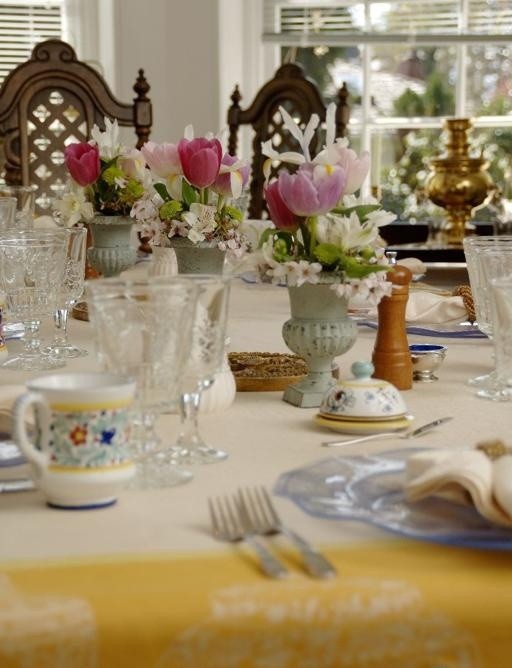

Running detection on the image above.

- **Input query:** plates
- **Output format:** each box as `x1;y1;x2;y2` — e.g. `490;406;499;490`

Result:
350;308;487;338
238;271;287;287
271;446;511;550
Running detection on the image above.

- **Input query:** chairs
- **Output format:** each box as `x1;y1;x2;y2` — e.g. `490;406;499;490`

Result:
1;39;156;218
220;62;348;222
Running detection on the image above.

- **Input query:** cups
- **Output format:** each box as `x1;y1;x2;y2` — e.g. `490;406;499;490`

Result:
10;372;137;512
11;227;88;361
474;246;512;401
82;276;194;490
463;235;512;388
0;238;67;372
145;273;233;466
1;187;39;230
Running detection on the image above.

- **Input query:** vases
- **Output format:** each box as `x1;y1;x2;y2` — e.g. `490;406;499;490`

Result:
281;285;354;408
166;238;225;275
425;116;490;246
83;213;136;276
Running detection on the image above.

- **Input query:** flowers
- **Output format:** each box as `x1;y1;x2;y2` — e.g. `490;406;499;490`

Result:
264;140;391;318
144;127;252;255
57;118;147;234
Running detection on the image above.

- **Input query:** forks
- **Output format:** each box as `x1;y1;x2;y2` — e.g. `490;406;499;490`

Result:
321;417;455;447
236;485;338;579
206;492;292;581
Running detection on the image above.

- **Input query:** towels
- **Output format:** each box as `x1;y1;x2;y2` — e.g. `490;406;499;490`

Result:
402;446;512;526
368;290;468;326
